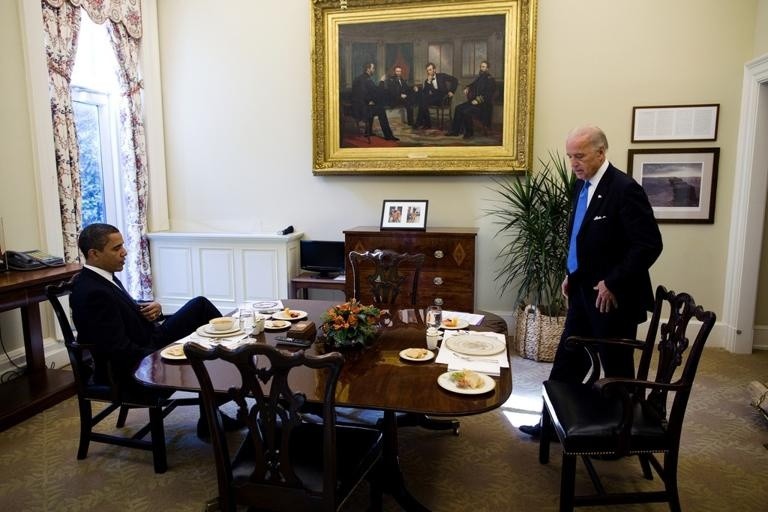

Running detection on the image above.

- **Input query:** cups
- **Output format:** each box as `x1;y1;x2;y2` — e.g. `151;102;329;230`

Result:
427;306;442;330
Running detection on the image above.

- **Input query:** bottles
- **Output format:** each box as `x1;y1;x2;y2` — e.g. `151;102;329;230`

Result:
252;315;266;335
426;328;439;350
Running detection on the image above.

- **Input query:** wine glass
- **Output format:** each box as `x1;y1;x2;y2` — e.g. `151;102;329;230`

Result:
240;301;255;344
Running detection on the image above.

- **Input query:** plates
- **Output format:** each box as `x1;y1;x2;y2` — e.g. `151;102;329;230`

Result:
203;324;240;334
438;319;469;329
399;348;434;365
444;333;507;356
159;345;191;361
195;323;247;338
438;369;496;396
263;307;309;330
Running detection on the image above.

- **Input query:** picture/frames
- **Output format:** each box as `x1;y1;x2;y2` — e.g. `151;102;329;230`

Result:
629;103;721;144
380;199;430;231
626;147;722;226
306;1;540;178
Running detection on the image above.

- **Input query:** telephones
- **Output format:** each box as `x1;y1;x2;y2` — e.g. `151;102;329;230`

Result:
2;249;63;270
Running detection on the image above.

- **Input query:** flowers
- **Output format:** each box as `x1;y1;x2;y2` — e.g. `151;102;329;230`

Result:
319;298;388;350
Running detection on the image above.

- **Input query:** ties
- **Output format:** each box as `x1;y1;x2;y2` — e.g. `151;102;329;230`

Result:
566;180;591;275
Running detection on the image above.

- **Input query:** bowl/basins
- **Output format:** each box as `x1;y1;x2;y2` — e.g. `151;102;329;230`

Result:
210;316;236;330
252;301;278;314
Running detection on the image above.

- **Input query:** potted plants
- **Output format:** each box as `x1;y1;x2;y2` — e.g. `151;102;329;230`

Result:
478;142;584;365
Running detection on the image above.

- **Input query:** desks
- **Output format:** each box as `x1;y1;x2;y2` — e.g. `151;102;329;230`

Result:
289;269;346;301
0;256;87;435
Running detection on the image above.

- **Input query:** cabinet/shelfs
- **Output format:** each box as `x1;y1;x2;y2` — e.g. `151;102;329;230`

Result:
341;222;481;315
144;228;306;325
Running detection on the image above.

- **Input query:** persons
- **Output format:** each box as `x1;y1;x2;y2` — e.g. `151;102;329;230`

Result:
352;63;400;141
445;62;496;140
389;206;401;224
388;66;416;126
415;63;457;131
409;208;417;223
518;120;663;443
69;224;237;432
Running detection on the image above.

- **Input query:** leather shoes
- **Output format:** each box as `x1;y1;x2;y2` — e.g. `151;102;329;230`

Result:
197;411;243;438
519;424;559;443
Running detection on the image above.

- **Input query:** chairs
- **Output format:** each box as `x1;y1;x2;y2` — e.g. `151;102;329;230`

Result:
534;284;719;512
40;270;202;477
181;338;393;512
344;245;430;314
